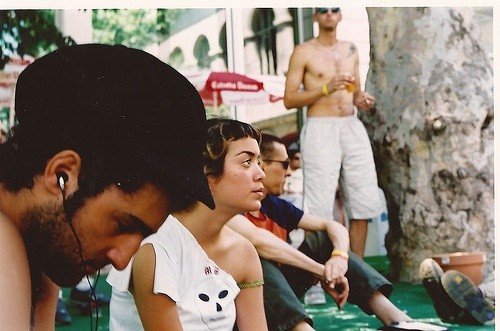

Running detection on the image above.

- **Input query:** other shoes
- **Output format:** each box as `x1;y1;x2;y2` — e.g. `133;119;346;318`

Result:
55;287;110;326
377;322;451;331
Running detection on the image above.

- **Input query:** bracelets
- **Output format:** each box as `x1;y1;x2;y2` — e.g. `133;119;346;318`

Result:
331;250;350;262
322;84;329;97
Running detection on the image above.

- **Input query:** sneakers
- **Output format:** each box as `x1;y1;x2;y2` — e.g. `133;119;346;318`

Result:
419;258;466;324
441;270;495;325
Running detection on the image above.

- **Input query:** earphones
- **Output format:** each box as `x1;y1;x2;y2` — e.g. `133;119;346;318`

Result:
56;171;68;192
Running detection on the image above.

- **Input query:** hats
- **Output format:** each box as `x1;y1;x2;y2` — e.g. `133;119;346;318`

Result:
15;44;216;211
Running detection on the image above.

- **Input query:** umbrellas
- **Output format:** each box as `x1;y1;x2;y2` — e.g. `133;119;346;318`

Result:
186;72;284;115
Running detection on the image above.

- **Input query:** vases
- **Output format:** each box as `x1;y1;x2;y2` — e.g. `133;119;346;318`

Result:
430;252;484;286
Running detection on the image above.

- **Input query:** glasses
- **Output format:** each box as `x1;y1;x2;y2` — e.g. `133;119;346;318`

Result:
315;8;341;13
260;159;289;170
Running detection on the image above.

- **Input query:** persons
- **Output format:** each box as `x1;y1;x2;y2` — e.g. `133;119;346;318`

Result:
282;8;383;305
0;43;495;331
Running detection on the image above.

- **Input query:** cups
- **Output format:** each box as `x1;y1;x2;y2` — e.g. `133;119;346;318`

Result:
345;74;360;94
